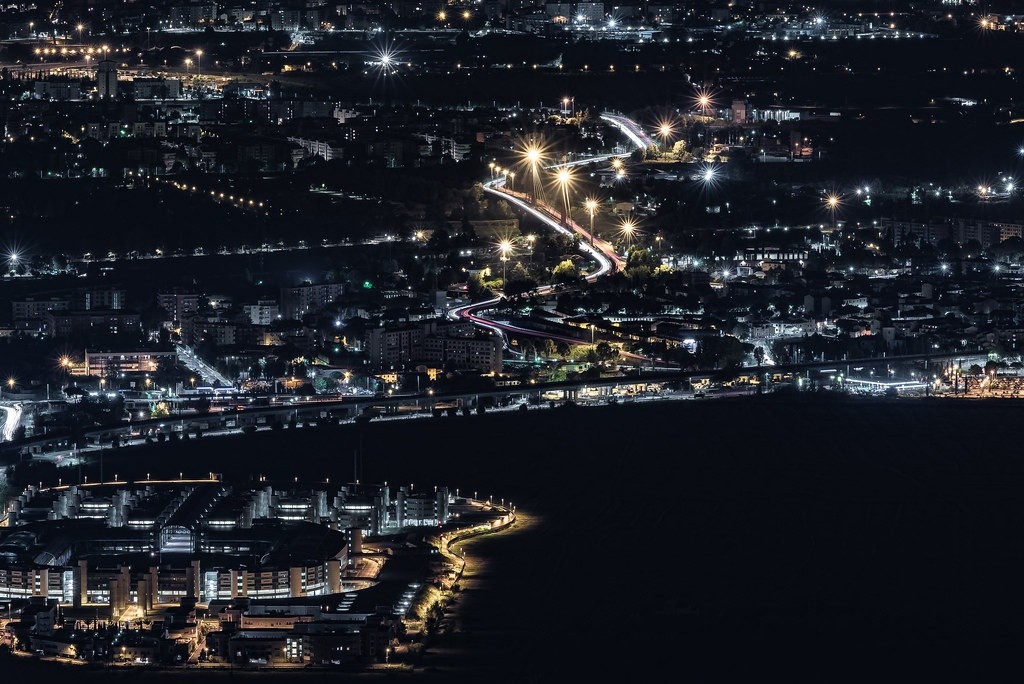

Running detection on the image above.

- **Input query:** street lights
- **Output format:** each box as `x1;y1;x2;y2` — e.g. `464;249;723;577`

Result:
197;49;202;74
623;223;633;249
559;171;572;227
662;123;670;162
700;94;709;123
500;239;511;293
830;197;838;231
528;234;536;270
528;149;541;206
586;200;599;246
